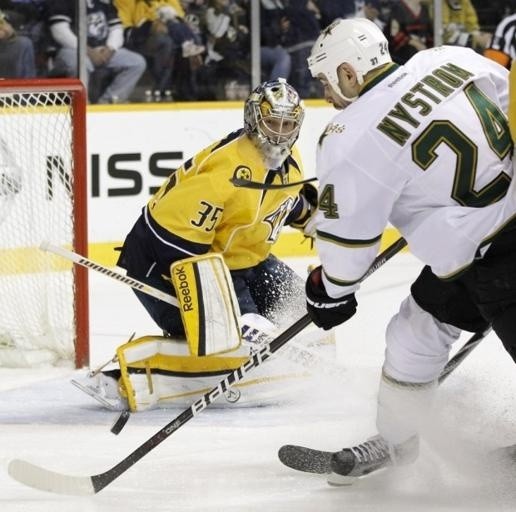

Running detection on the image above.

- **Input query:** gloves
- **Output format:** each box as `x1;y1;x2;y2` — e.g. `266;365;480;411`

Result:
306;266;358;331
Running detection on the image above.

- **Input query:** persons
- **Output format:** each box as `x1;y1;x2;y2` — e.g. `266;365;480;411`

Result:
305;17;516;479
0;0;516;103
76;78;317;410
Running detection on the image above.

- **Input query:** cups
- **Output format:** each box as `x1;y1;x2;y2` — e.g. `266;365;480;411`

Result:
223;82;250;100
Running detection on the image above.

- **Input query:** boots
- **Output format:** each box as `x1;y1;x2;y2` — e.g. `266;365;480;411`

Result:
88;368;131;406
331;435;421;477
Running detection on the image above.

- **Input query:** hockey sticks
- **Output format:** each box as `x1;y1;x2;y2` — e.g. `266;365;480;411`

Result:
279;326;492;474
8;237;408;495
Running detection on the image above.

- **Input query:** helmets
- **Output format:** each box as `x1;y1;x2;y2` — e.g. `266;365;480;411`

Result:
244;79;304;144
307;17;391;104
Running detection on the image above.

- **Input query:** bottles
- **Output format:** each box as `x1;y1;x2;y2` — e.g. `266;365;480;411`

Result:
144;89;174;103
